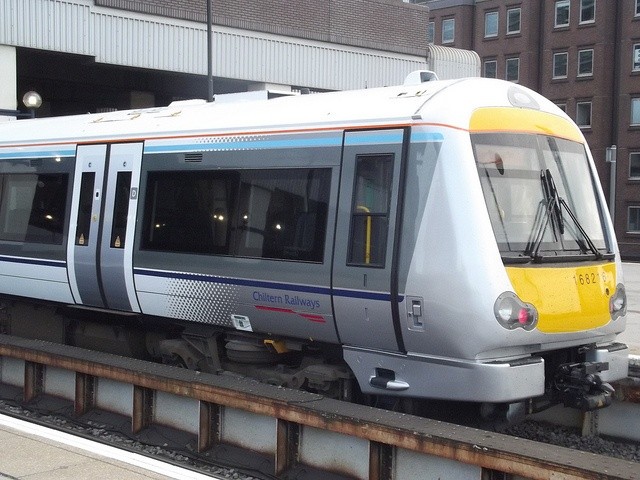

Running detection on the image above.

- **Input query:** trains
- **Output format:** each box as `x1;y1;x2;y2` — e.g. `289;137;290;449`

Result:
0;69;628;426
